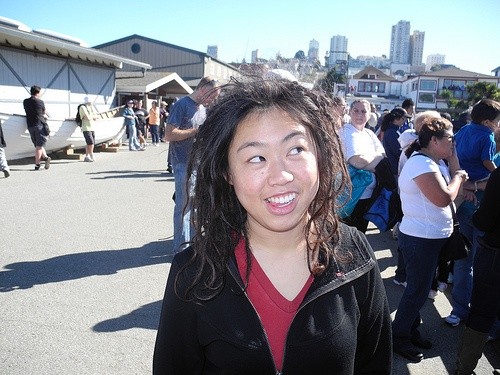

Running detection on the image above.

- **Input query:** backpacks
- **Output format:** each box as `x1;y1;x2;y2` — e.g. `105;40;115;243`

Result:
75;104;90;127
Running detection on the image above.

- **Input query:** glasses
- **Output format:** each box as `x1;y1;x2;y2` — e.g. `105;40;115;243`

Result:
435;132;458;143
400;117;408;123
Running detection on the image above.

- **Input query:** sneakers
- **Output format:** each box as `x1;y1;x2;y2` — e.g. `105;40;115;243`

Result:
129;146;137;152
44;157;51;169
392;278;408;288
3;168;11;178
438;281;447;292
444;312;461;328
447;271;454;284
427;290;439;300
411;331;428;354
394;338;424;364
84;157;94;162
34;163;40;171
89;154;95;161
138;146;144;152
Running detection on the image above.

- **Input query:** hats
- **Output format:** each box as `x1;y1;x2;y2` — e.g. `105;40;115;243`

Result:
160;102;170;107
127;99;135;104
83;95;95;104
390;106;412;119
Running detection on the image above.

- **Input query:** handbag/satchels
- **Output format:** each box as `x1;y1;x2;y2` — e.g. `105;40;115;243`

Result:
438;226;473;264
43;123;51;136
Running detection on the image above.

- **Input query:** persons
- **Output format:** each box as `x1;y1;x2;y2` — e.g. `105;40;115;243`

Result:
132;100;150;152
400;97;476;195
443;162;500;375
151;73;394;375
0;117;10;178
381;107;413;238
170;96;180;112
131;99;139;113
166;76;220;256
365;102;381;134
159;101;169;143
23;85;51;171
122;99;146;152
391;117;469;362
328;91;345;128
78;96;97;163
451;98;500;182
335;98;387;237
447;121;500;343
144;100;161;146
